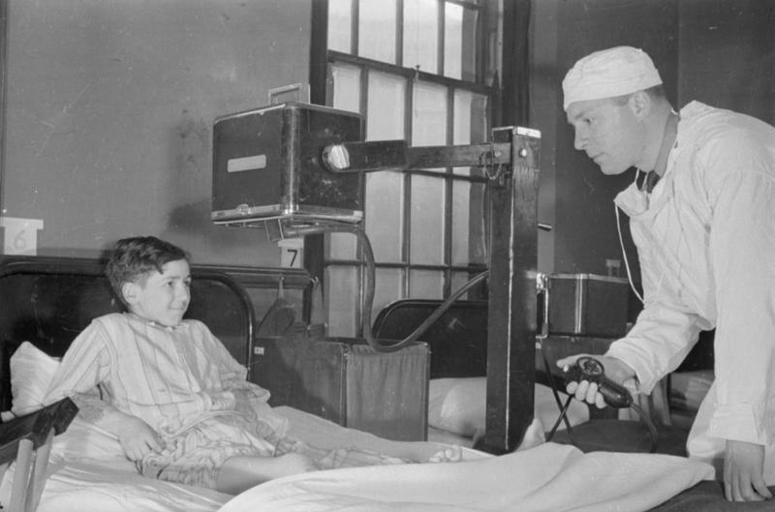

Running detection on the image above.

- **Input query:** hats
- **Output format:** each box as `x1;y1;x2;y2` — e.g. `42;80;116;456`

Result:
563;45;664;113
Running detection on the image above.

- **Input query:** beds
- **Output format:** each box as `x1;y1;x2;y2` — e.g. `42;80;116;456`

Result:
2;255;775;510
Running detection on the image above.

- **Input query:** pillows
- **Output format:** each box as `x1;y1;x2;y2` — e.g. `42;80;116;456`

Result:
1;341;272;425
427;376;643;438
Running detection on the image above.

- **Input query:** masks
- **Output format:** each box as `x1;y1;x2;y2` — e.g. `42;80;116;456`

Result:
614;108;679;229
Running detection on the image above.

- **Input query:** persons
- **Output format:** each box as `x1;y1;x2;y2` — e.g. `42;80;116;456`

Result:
39;234;548;495
552;43;774;506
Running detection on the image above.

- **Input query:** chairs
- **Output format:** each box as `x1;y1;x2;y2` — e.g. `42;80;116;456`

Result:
370;298;591;446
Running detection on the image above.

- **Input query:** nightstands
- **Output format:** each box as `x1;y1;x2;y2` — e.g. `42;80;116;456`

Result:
254;334;433;445
